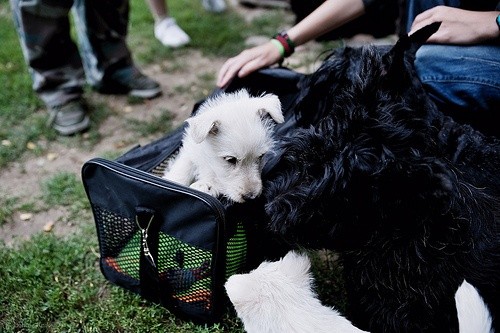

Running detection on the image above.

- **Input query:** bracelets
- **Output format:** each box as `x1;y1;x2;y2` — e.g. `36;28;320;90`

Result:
270;32;295;61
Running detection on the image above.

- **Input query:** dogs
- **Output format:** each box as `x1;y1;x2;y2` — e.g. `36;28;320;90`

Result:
159;87;285;203
294;19;500;199
260;115;500;333
223;250;494;333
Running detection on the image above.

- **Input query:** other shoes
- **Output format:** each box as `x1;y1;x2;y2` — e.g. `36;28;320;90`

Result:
47;96;90;136
98;68;162;99
202;0;226;13
154;17;191;48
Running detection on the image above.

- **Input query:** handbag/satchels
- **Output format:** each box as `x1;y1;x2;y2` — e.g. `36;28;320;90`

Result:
80;64;307;325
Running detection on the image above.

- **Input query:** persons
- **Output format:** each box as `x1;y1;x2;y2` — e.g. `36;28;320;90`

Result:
9;0;162;135
215;0;500;121
147;0;228;46
